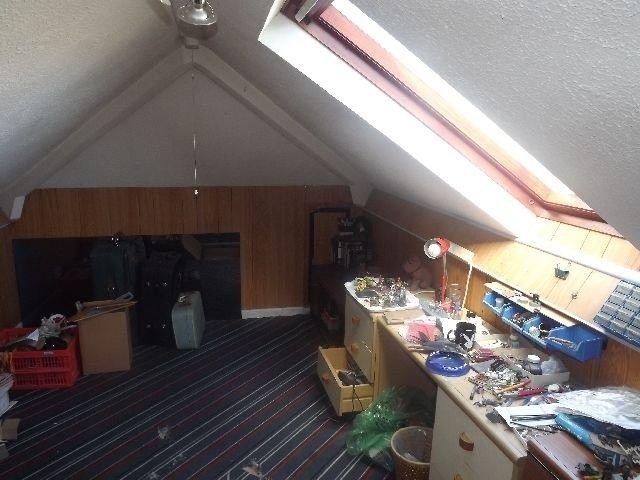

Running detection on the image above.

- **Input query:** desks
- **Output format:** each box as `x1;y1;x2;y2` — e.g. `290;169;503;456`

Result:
374;312;598;480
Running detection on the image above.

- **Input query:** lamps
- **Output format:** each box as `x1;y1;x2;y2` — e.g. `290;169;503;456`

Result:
424;237;450;305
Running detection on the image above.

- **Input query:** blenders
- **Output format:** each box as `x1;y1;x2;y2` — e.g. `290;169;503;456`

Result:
330;215;358;266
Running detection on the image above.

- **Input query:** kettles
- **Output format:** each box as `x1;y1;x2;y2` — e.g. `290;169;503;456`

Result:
344;243;371;276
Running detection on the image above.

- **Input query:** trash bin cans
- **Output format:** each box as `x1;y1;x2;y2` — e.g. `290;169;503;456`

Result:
390;425;432;475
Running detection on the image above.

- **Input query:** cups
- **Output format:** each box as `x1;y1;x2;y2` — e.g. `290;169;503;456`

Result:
447;322;476;350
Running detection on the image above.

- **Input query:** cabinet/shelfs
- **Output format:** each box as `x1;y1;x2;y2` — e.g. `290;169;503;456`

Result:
316;280;376;415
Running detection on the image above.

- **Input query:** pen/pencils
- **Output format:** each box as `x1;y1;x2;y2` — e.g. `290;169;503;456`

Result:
496;379;538;395
510;413;559;421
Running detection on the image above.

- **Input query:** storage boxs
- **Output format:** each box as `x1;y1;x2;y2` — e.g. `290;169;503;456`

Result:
79;299;134;377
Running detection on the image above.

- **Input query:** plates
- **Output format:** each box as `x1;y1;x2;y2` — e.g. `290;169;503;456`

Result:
425;350;472;377
